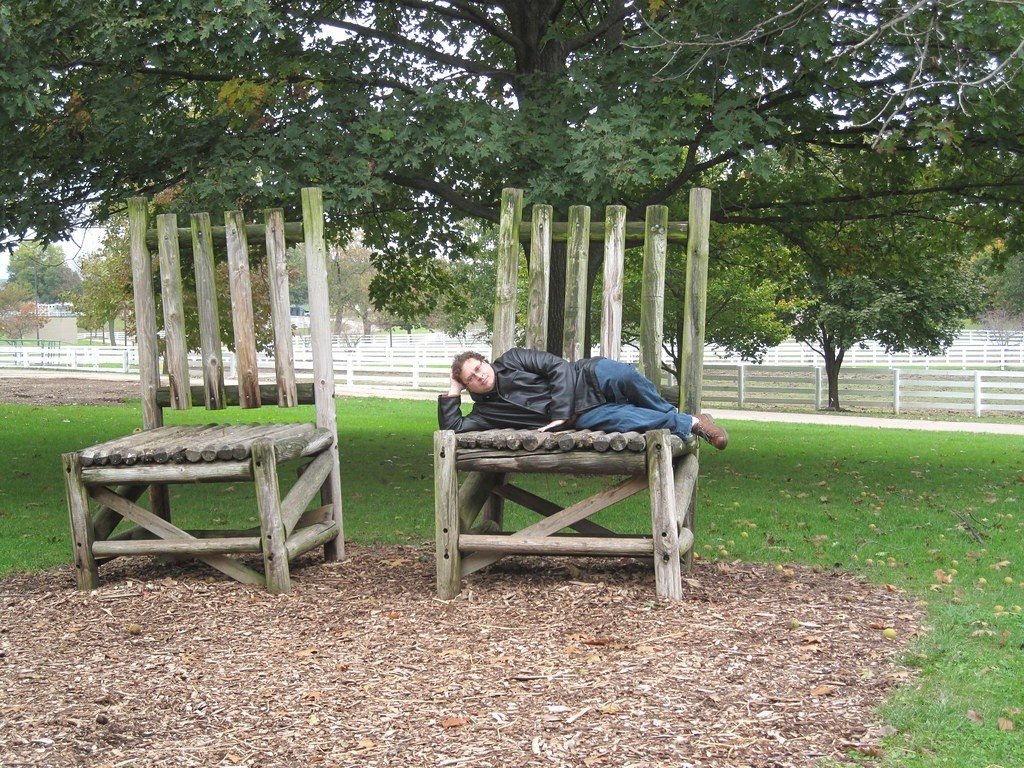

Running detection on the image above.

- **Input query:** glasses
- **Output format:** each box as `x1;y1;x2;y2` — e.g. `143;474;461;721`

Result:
465;361;483;388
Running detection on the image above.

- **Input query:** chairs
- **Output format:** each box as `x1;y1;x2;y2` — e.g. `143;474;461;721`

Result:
433;183;717;601
55;187;345;596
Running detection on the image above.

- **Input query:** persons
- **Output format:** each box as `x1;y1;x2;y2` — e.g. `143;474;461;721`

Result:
438;347;728;451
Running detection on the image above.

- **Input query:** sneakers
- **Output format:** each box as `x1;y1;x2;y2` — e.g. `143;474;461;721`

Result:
691;413;728;450
